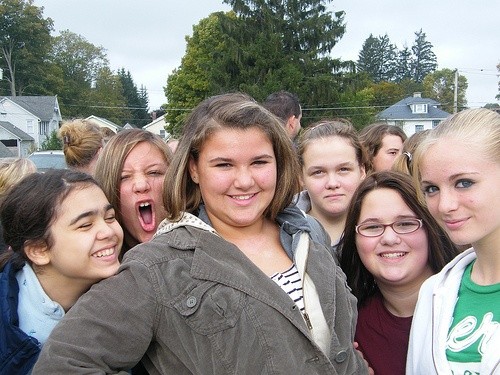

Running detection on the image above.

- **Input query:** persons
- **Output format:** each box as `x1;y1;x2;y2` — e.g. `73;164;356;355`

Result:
31;92;370;375
93;128;175;254
58;119;117;176
333;170;457;375
263;90;302;140
166;138;181;154
0;169;124;375
391;129;432;175
0;156;37;205
360;123;408;173
406;110;500;375
290;118;372;254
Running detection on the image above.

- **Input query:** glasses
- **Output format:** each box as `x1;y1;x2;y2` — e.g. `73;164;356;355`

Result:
354;217;423;238
